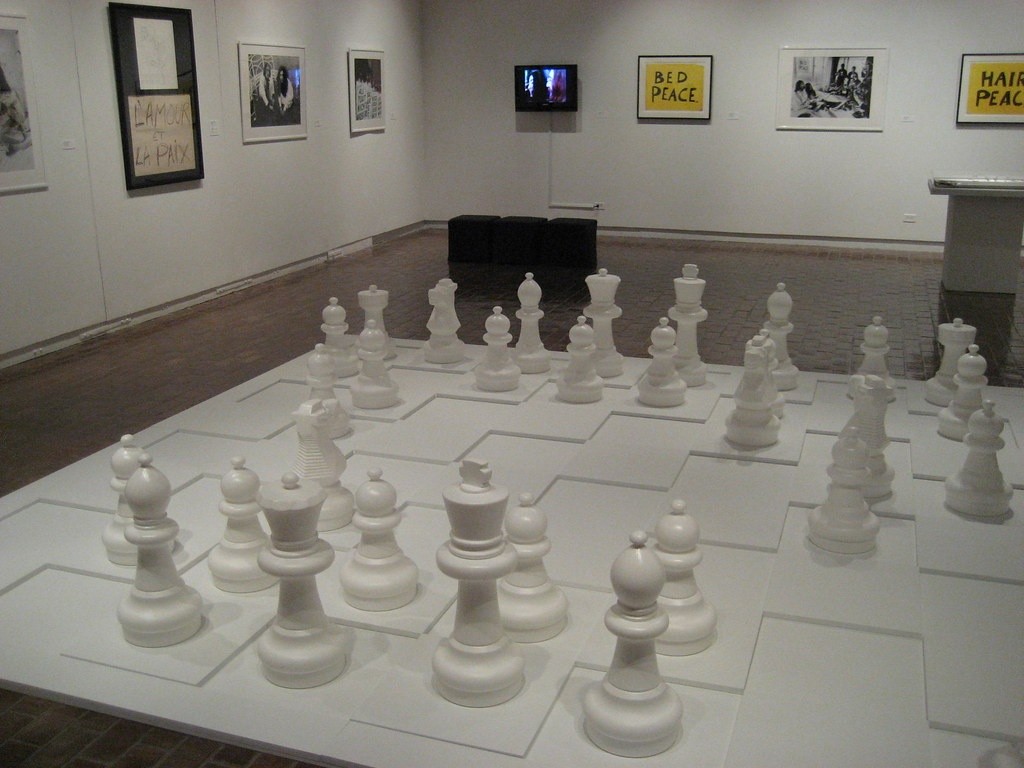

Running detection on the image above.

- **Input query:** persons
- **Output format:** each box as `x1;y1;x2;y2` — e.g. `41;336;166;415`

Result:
791;56;873;119
258;63;276;111
275;65;293;114
546;69;567;102
528;72;545;101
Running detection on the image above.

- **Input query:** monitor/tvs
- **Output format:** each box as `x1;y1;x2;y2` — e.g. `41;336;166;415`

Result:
515;64;577;113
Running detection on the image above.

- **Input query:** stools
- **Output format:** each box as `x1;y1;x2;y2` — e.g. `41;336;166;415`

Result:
546;217;597;271
447;214;500;262
491;216;550;264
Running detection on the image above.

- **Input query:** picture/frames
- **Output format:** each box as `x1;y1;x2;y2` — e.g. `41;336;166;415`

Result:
348;48;386;134
775;47;889;133
0;14;48;195
238;41;308;144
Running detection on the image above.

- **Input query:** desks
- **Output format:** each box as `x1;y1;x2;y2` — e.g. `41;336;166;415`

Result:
928;176;1024;291
0;334;1021;768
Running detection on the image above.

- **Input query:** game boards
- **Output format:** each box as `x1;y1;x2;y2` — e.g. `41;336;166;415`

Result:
0;332;1023;768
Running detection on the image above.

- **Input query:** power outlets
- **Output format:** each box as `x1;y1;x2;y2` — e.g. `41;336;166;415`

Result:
594;202;605;209
904;214;916;223
209;120;218;136
